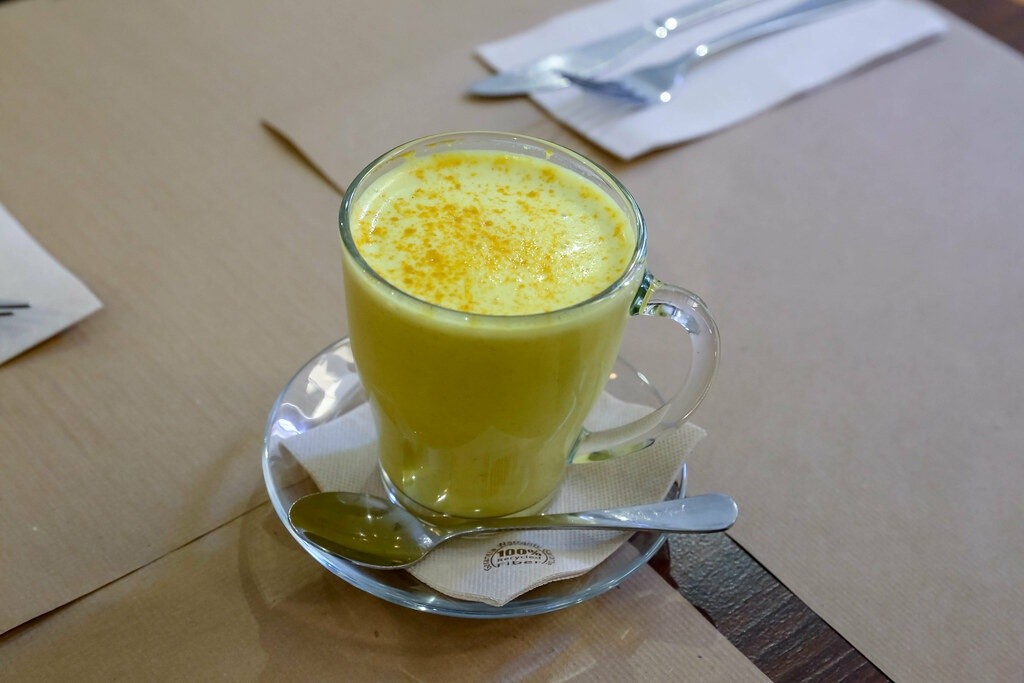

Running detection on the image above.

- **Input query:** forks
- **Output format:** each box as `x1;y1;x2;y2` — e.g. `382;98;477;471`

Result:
552;1;872;108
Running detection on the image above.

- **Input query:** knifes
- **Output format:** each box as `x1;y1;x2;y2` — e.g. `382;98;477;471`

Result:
468;1;752;99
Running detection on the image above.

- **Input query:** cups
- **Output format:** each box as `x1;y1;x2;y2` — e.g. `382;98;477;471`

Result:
338;132;724;526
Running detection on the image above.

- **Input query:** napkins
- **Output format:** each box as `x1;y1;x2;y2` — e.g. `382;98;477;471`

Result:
0;206;104;368
472;0;954;162
279;390;706;608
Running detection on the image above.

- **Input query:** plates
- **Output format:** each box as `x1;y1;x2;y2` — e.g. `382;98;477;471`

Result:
260;334;692;617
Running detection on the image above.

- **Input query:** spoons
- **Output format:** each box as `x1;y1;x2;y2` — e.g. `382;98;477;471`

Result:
284;491;740;567
